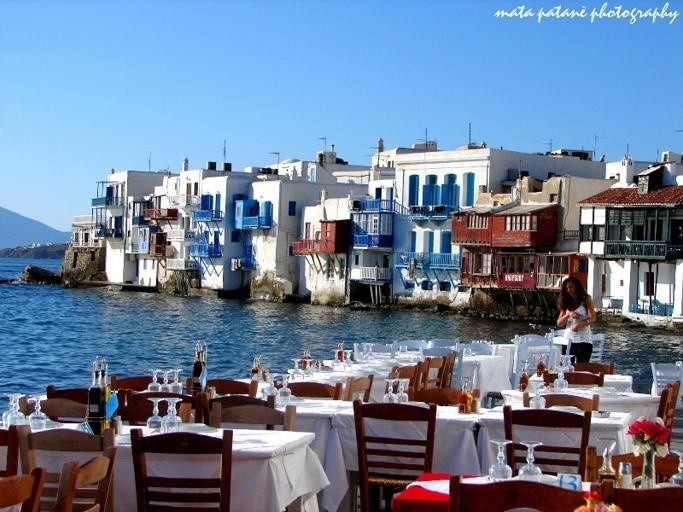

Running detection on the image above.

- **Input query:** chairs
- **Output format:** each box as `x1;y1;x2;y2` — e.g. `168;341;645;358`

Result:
0;467;47;512
205;391;276;430
510;334;555;386
573;362;614;375
352;397;439;512
417;357;428;394
456;338;495;356
391;365;420;402
649;360;682;409
394;339;428;351
551;329;567;335
43;383;124;423
591;334;605;362
521;391;610;415
23;423;113;510
183;379;259;396
125;387;204;424
109;374;195;395
504;404;593;485
0;424;19;476
657;380;683;446
272;379;342;401
130;428;234;512
448;474;589;511
344;375;374;402
53;447;117;511
211;403;296;431
426;356;439;391
587;445;680;484
599;479;683;512
444;350;458;389
427;337;462;347
412;385;481;411
543;371;604;388
352;340;393;354
421;348;462;389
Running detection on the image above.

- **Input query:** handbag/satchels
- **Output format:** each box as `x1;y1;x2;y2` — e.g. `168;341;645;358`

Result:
572;314;590;333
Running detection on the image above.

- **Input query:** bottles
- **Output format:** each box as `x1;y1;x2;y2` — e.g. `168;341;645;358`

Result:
598;446;615;481
459;387;473;411
100;369;110;428
89;370;102;434
617;462;631;488
199;351;205;368
192;352;204;395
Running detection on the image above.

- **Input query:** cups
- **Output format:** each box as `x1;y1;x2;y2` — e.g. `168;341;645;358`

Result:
669;450;683;487
262;373;280;403
146;398;164;434
302;359;314;379
148;369;161;391
170;369;183;394
488;439;513;480
554;367;568;393
2;392;21;429
290;358;304;380
8;394;25;428
277;374;291;404
331;349;340;367
519;441;543;481
529;381;546;409
159;371;170;392
560;355;575;372
361;343;375;361
29;395;46;430
344;350;352;367
383;379;398;404
463;348;471;359
161;398;183;433
395;379;410;403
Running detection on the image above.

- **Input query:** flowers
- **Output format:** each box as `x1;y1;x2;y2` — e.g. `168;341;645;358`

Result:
626;416;671;458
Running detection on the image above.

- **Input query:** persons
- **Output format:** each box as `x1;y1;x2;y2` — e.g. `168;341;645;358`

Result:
557;277;596;363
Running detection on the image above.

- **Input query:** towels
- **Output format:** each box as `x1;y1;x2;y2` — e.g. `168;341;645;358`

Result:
405;475;593;495
